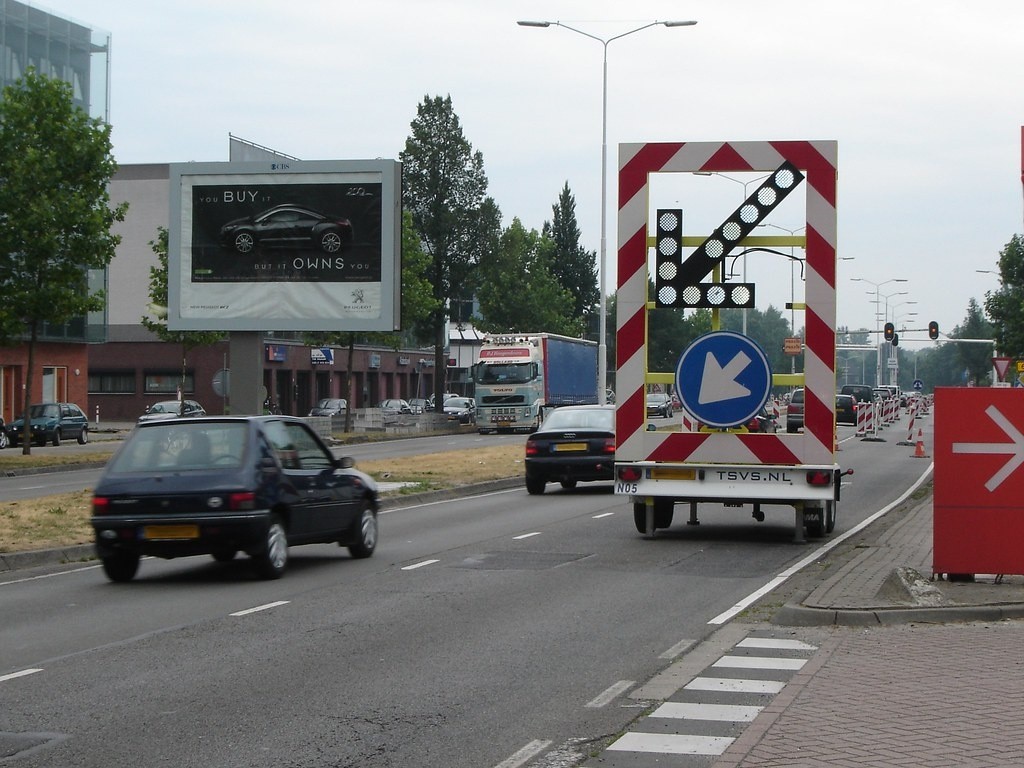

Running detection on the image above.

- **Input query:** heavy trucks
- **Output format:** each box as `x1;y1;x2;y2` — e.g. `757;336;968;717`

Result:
474;331;599;434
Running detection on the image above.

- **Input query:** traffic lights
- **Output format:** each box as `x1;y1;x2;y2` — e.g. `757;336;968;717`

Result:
448;359;457;366
892;335;899;346
929;322;939;339
884;323;895;341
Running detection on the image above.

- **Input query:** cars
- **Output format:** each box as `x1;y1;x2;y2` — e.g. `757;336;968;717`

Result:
697;406;778;433
787;389;804;433
138;400;208;422
308;398;357;418
524;405;617;494
410;391;476;423
223;205;355;259
646;393;674;417
0;404;89;448
372;398;414;414
88;415;381;584
835;385;921;426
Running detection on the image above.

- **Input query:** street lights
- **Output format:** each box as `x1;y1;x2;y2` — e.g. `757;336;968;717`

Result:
848;276;919;386
518;20;696;406
975;269;1010;352
693;171;776;336
757;222;805;373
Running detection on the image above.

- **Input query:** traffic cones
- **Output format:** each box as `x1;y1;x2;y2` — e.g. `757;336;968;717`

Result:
910;428;930;458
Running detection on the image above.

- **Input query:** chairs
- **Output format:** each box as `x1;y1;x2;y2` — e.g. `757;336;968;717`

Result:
177;448;210;465
